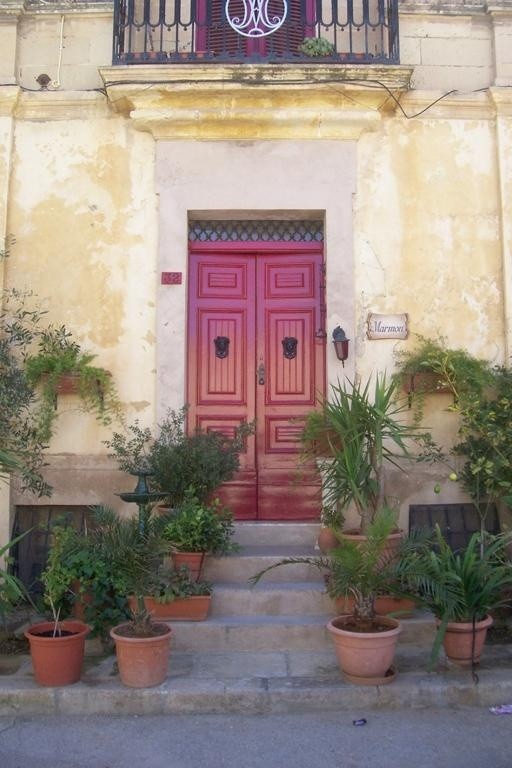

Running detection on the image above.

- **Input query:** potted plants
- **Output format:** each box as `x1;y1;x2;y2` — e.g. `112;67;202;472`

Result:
396;349;480;395
25;325;116;424
23;371;512;688
